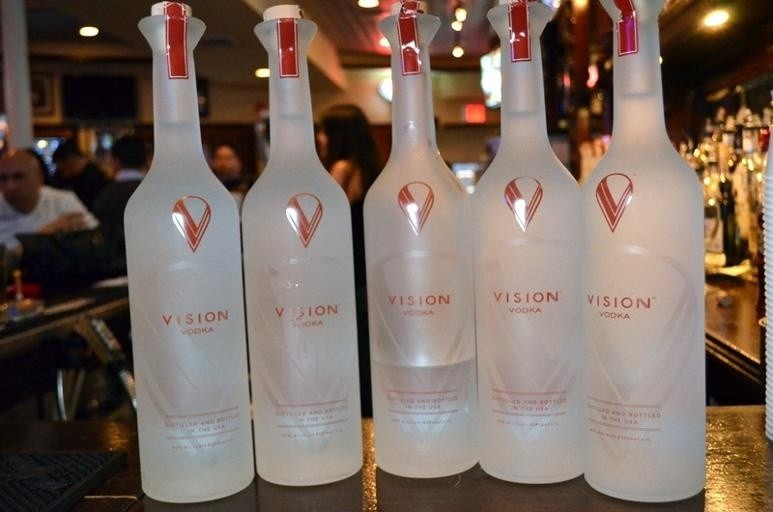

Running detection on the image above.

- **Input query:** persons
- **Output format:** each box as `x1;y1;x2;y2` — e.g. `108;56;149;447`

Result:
0;104;383;418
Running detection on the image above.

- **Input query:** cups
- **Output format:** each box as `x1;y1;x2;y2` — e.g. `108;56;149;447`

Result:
762;128;773;438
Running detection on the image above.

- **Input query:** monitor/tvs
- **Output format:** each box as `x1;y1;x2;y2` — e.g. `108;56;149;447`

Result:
478;47;502;110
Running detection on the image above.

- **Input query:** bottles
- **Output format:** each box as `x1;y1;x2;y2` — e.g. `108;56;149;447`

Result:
361;3;481;485
122;1;256;503
577;105;768;280
471;0;588;488
243;3;365;490
580;0;707;502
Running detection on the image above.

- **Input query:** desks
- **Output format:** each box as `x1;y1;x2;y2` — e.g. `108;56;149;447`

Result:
706;276;764;395
0;277;136;421
